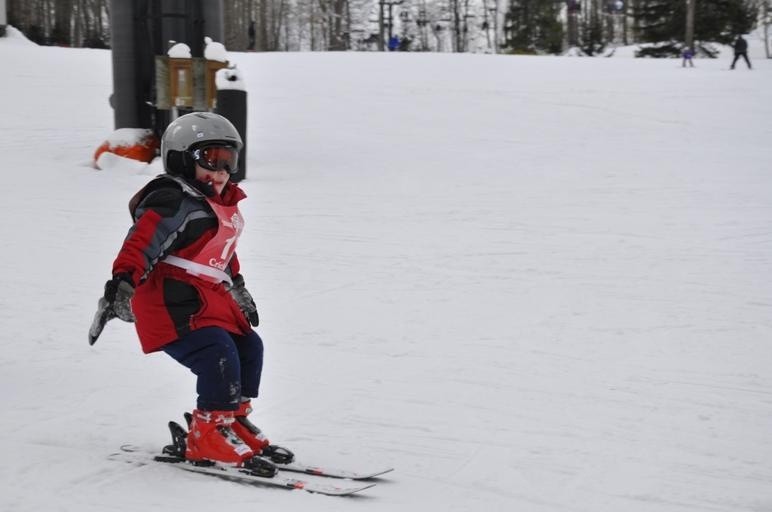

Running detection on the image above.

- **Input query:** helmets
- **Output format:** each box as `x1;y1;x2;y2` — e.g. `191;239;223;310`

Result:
161;112;244;179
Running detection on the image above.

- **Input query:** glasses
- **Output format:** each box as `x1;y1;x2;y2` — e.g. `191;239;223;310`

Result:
189;143;239;174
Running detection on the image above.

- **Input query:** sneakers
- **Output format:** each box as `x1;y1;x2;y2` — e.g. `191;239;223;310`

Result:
183;402;269;461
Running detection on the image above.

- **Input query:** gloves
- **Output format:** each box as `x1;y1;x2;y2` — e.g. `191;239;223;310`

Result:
223;274;259;327
88;276;136;344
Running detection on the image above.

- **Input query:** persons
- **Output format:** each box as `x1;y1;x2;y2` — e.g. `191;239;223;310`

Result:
398;37;412;52
85;109;297;478
387;33;399;50
729;33;752;70
680;44;695;66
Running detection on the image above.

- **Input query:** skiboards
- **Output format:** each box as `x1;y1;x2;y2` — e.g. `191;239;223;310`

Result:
105;444;396;496
